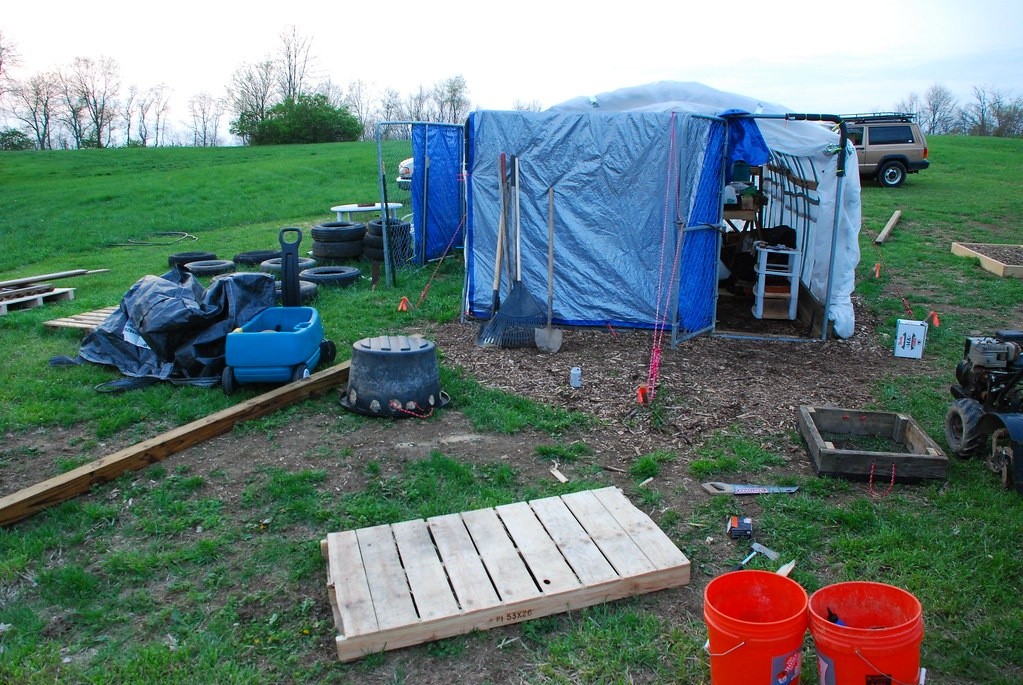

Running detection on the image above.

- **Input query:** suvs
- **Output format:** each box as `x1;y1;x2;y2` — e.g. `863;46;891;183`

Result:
835;114;930;187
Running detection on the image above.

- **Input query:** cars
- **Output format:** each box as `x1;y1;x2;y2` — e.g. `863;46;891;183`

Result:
396;156;414;191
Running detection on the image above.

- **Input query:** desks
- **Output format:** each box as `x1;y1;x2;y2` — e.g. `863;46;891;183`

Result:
330;203;403;223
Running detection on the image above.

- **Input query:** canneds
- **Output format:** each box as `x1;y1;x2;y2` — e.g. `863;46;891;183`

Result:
570;367;581;387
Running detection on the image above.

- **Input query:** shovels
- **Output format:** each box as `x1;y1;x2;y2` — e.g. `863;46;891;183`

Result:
474;211;503;347
534;188;562;354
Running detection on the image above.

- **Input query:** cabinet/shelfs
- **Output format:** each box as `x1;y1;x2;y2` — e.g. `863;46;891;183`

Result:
751;243;802;322
